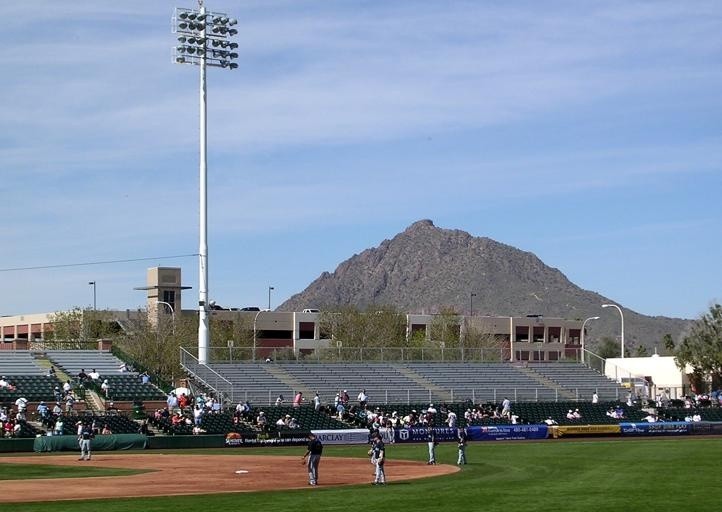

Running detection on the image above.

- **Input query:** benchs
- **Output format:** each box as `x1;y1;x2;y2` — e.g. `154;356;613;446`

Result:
184;361;636;406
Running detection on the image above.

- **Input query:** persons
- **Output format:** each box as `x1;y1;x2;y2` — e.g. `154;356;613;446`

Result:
275;395;283;405
541;415;557;425
276;415;299;428
0;365;114;462
301;434;323;485
314;393;321;411
566;388;659;424
138;420;147;433
369;429;383;476
426;424;438;466
226;429;240;443
233;401;249;423
140;403;146;411
335;390;457;428
456;425;468;465
464;397;519;425
257;412;266;425
370;435;390;485
155;392;230;435
294;392;302;406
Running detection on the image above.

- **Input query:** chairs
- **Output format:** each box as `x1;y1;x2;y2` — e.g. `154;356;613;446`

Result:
2;374;722;436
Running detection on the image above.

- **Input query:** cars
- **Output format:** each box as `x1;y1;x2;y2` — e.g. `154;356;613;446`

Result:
303;309;320;313
229;307;260;311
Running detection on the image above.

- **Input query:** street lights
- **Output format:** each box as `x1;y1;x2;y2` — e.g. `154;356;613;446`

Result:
581;317;601;363
471;292;477;316
252;309;272;360
155;300;175;386
269;286;274;309
601;304;624;358
89;281;96;312
169;0;238;365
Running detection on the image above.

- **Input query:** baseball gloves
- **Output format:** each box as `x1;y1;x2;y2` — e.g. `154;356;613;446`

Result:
89;435;96;439
301;457;306;465
